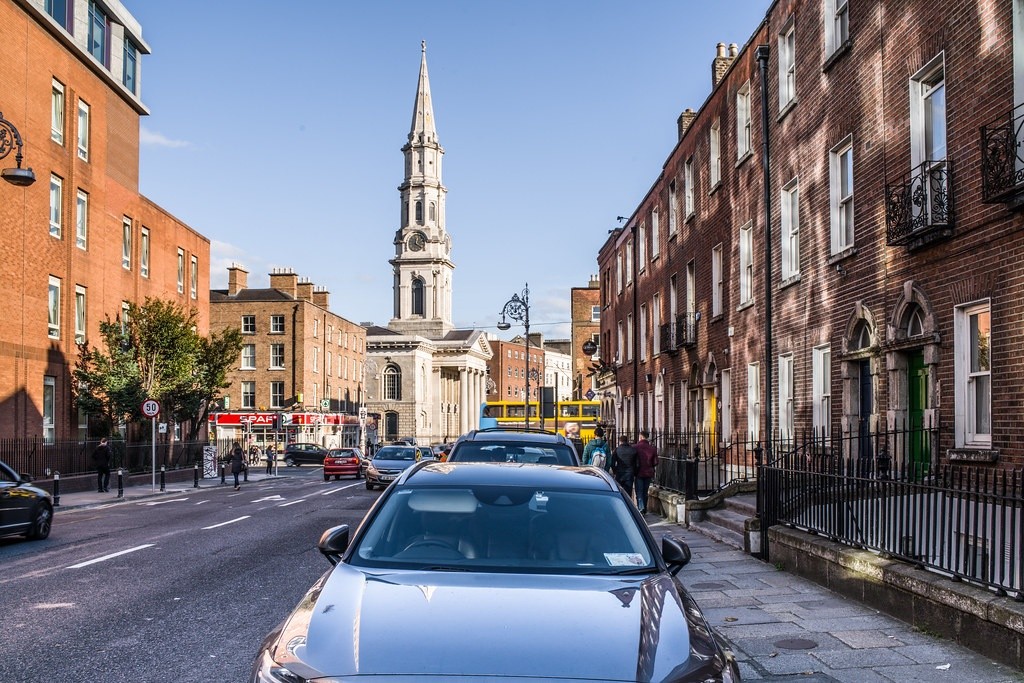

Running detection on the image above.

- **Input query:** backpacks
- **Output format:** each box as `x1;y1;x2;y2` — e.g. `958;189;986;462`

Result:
588;444;606;470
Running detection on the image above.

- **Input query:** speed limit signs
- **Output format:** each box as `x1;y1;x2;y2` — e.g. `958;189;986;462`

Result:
140;399;160;418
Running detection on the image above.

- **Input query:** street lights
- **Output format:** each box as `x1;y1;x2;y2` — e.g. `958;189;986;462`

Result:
498;283;530;431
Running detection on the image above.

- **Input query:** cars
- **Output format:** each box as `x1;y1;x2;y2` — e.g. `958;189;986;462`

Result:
0;461;54;539
365;442;448;490
323;447;370;481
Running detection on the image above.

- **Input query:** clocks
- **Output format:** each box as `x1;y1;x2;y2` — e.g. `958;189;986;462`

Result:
409;234;425;252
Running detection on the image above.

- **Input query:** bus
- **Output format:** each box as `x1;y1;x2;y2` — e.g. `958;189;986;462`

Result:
479;401;602;446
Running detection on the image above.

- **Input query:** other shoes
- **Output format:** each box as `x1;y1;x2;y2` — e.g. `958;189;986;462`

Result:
104;488;109;492
234;485;241;490
98;489;104;492
639;509;648;515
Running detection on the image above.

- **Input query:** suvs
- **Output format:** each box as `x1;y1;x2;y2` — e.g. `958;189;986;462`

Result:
445;428;581;466
283;443;329;467
246;461;741;683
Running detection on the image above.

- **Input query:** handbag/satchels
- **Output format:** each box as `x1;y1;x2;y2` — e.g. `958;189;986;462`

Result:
242;460;246;470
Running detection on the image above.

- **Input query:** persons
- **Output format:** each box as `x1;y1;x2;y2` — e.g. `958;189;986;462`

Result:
249;445;262;465
226;442;245;490
266;445;275;476
416;450;421;461
439;452;447;461
582;427;611;473
93;438;111;492
633;431;659;515
367;438;373;447
611;435;637;497
565;423;580;437
444;436;448;444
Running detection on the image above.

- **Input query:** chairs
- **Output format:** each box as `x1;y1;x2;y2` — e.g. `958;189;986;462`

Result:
528;497;610;562
461;449;494;461
402;513;480;563
539;455;558;464
